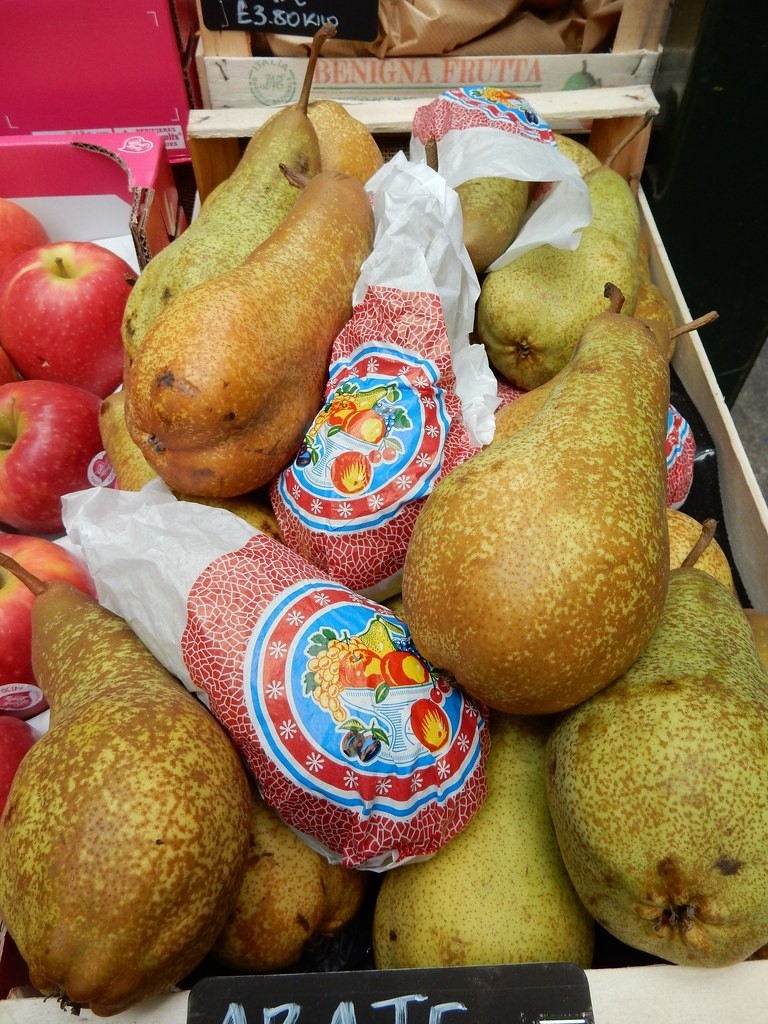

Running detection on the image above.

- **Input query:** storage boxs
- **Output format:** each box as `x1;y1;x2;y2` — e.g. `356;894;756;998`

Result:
0;0;768;1024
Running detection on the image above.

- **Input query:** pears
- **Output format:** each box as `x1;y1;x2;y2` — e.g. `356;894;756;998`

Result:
1;24;768;1017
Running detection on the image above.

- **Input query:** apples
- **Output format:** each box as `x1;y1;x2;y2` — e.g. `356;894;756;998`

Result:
0;196;51;274
0;715;41;817
0;380;115;534
0;531;98;721
0;242;140;398
